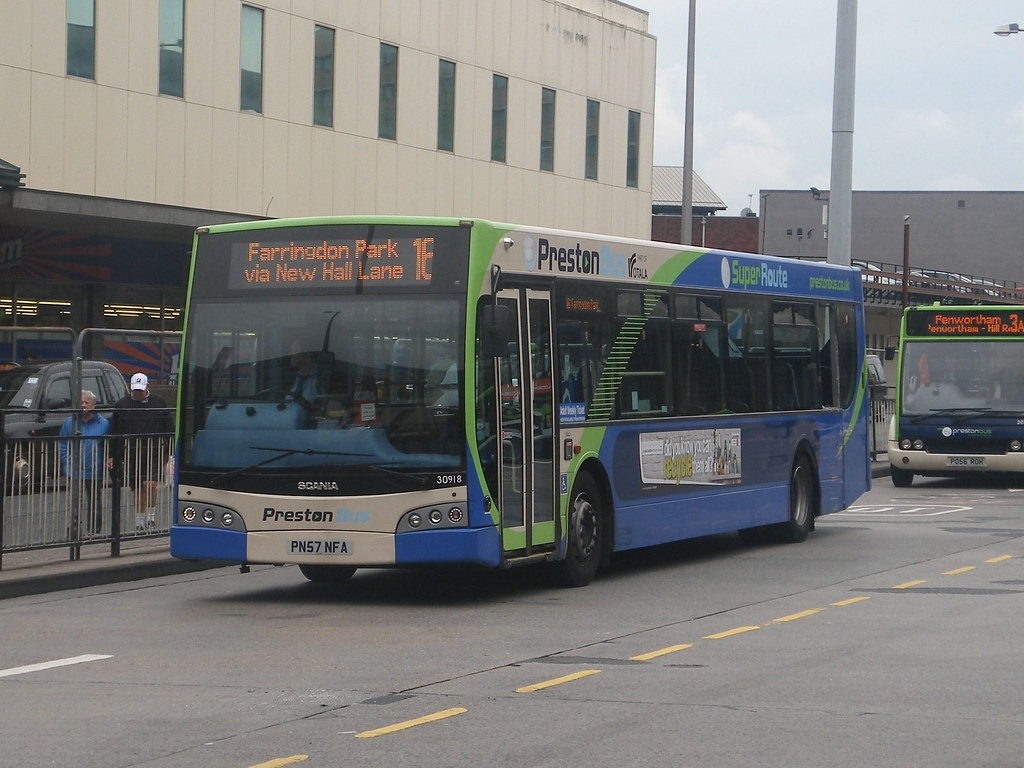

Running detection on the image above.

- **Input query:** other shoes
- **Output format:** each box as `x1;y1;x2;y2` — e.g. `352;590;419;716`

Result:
147;520;156;530
136;525;146;535
88;528;98;535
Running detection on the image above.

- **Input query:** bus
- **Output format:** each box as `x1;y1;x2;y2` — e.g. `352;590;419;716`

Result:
887;301;1024;491
168;214;872;587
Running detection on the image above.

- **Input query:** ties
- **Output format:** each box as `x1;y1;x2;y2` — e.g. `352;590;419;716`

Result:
293;372;311;395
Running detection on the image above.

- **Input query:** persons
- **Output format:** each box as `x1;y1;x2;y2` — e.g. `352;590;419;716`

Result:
106;372;176;533
58;389;111;539
264;338;343;430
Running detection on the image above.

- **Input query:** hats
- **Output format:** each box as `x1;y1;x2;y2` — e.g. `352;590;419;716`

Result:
131;373;148;390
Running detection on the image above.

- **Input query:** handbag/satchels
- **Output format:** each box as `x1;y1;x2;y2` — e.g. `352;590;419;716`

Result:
164;454;175;487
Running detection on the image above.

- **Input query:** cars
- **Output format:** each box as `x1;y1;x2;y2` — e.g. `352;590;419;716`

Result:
866;354;887;423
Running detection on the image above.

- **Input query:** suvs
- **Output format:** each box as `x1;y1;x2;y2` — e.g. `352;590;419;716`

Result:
0;360;130;494
